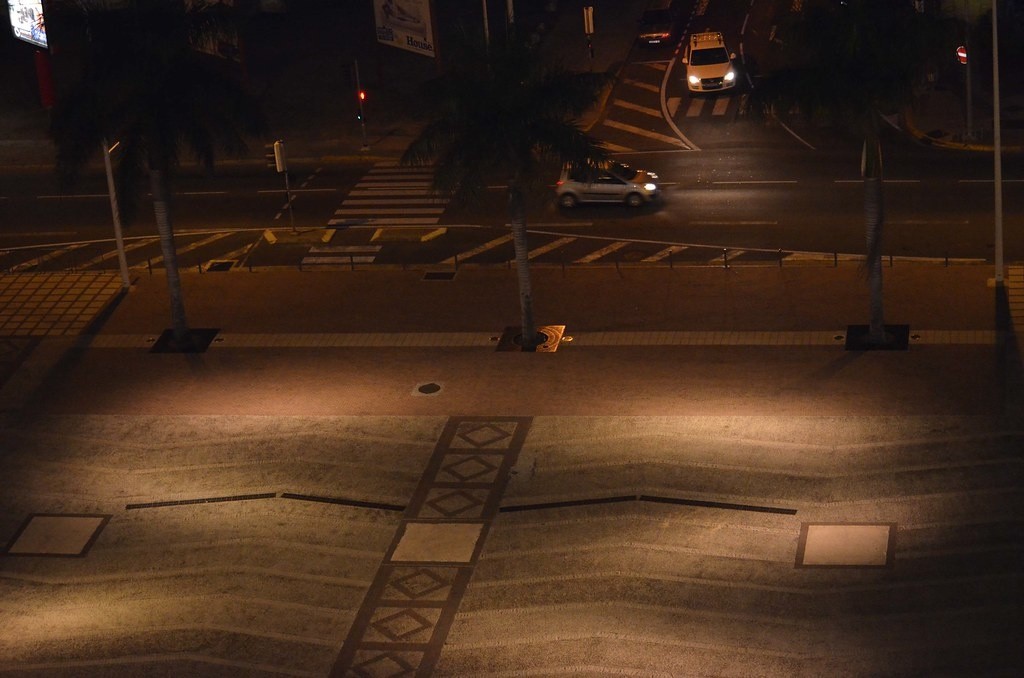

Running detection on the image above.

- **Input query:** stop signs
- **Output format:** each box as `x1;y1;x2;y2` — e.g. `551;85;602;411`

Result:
956;46;967;64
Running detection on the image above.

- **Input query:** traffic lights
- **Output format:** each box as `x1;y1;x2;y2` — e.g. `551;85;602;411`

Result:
263;143;277;169
584;38;592;49
360;92;365;100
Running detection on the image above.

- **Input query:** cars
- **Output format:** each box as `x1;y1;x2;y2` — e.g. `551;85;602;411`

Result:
557;159;661;212
638;8;679;48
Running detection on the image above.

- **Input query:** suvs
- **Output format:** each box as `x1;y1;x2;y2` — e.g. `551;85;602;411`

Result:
682;31;737;92
